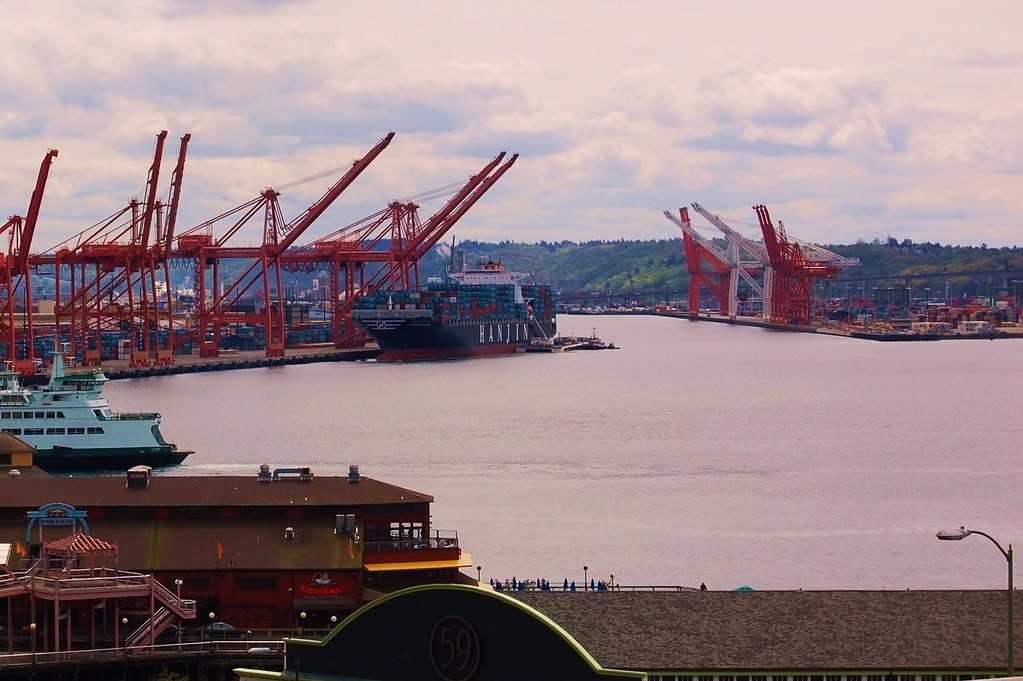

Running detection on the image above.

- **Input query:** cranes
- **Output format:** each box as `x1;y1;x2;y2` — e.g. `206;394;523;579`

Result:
0;148;60;378
57;128;523;368
662;203;862;330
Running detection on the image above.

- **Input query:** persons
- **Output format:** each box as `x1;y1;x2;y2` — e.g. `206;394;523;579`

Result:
490;576;608;592
700;582;707;591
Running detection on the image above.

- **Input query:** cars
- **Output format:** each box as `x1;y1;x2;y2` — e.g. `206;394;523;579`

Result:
194;621;254;641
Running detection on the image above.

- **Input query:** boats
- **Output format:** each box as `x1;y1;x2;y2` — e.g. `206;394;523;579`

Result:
0;463;474;639
0;347;197;469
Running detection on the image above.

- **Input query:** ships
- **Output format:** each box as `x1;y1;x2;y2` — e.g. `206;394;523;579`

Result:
348;235;557;363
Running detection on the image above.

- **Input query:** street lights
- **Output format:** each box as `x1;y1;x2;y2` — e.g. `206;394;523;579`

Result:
583;565;589;590
609;574;615;592
122;617;128;678
476;565;482;583
331;615;337;631
935;523;1016;678
208;612;215;654
175;579;183;654
30;623;38;673
299;611;307;641
